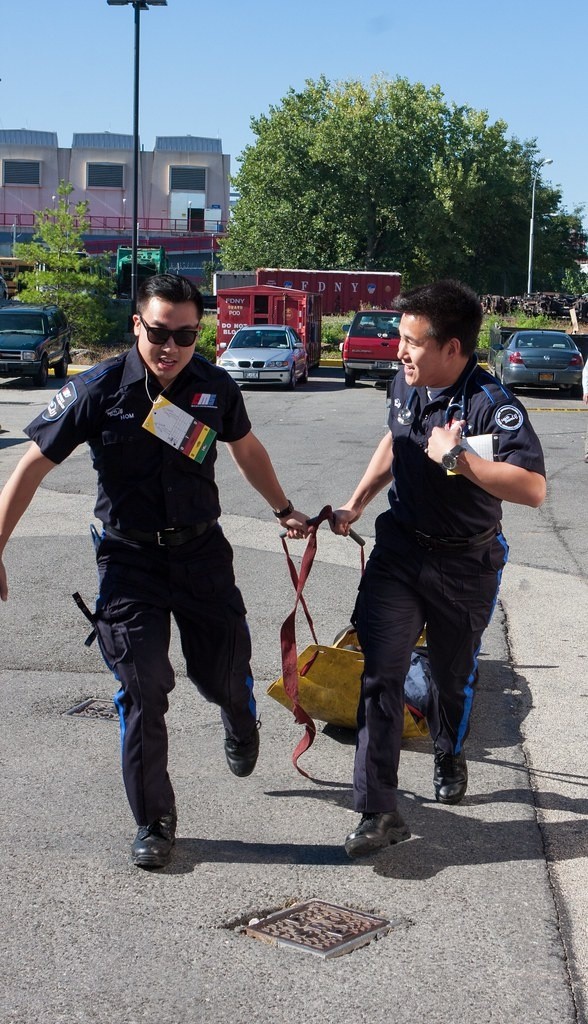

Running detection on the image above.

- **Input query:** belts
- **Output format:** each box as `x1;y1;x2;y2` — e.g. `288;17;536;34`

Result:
102;517;218;548
394;515;502;551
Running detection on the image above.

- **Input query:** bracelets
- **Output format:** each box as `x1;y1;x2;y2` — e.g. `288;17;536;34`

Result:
272;500;295;519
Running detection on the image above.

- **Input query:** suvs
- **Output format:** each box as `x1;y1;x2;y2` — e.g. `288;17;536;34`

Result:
0;300;72;388
342;309;406;388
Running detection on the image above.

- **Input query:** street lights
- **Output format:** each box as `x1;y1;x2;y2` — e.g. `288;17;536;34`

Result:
528;158;554;297
188;200;193;237
50;195;56;224
121;198;127;229
107;0;168;336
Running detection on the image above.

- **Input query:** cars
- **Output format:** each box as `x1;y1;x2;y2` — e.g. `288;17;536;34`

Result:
491;330;585;397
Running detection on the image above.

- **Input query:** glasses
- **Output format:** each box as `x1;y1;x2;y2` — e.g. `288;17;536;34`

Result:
137;310;201;347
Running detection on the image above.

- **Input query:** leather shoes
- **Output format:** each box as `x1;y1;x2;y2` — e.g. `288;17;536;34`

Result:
131;804;177;867
432;740;469;804
223;714;263;777
345;809;412;860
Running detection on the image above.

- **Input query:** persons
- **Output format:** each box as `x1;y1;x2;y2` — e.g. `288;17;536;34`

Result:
0;273;312;868
327;280;546;859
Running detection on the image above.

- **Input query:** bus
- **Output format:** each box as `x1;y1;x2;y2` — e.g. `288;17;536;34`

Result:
216;324;309;391
0;256;39;298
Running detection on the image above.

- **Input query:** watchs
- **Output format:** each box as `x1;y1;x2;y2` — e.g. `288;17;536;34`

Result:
441;445;467;471
